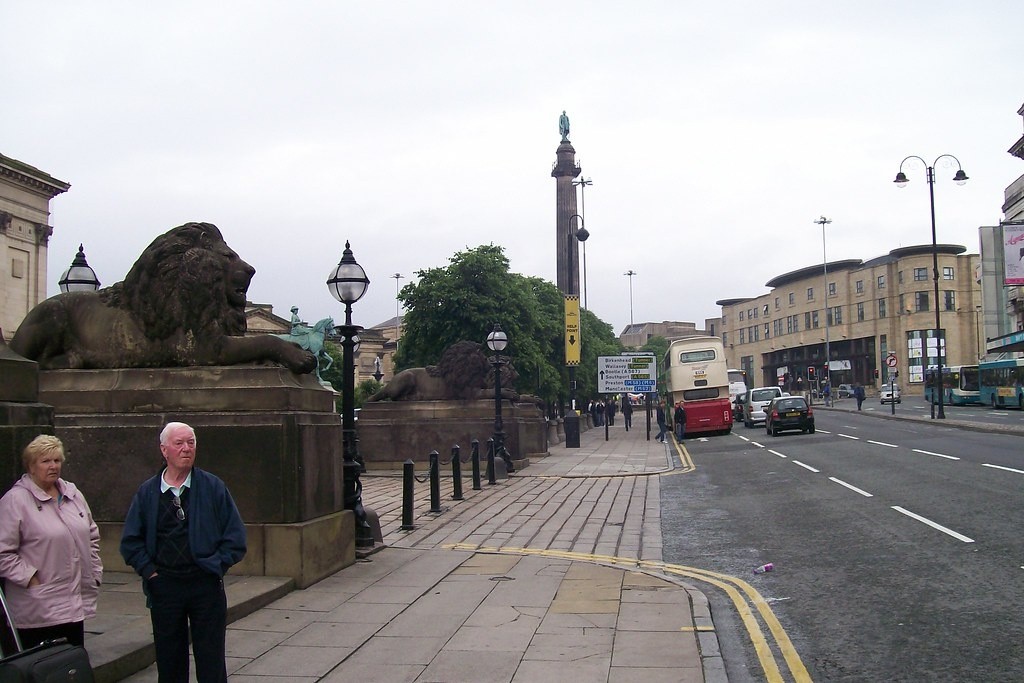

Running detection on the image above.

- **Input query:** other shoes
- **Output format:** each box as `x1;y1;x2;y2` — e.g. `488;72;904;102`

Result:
661;441;668;444
654;438;661;444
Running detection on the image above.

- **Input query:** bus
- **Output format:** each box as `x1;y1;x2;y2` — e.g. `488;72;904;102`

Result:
978;359;1024;409
727;369;748;410
656;336;734;434
924;365;980;406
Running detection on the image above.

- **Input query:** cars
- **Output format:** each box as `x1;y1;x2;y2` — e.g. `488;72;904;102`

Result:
732;393;746;422
763;396;815;436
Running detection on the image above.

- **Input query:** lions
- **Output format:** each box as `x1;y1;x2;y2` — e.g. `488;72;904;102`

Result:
10;221;319;375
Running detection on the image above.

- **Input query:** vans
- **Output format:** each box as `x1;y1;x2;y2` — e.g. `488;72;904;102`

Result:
878;384;902;404
742;387;783;428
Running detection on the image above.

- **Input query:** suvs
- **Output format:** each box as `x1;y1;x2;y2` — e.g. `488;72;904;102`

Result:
838;384;857;398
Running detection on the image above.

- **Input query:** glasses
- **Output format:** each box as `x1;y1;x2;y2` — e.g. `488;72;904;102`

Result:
171;496;185;521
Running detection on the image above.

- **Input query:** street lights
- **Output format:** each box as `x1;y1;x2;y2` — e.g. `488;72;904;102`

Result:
623;270;637;346
892;154;970;418
814;216;833;403
485;320;515;472
571;175;594;316
326;239;374;547
391;272;405;340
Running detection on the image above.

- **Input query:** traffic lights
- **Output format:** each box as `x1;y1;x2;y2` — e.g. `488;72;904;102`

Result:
875;369;878;379
808;367;815;381
823;364;828;380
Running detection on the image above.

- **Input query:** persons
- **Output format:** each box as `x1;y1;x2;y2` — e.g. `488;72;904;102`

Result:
1012;377;1018;386
0;434;104;658
822;382;831;407
675;400;686;444
120;421;247;683
588;398;605;426
854;381;865;411
607;397;616;426
622;399;633;431
290;305;310;336
655;400;668;444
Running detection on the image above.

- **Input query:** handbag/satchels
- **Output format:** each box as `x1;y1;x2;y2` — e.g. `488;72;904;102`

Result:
859;393;866;401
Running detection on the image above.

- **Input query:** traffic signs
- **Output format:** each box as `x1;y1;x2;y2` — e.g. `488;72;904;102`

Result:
598;355;656;393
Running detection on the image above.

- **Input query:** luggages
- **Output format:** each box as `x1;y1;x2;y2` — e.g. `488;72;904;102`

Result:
0;586;94;683
